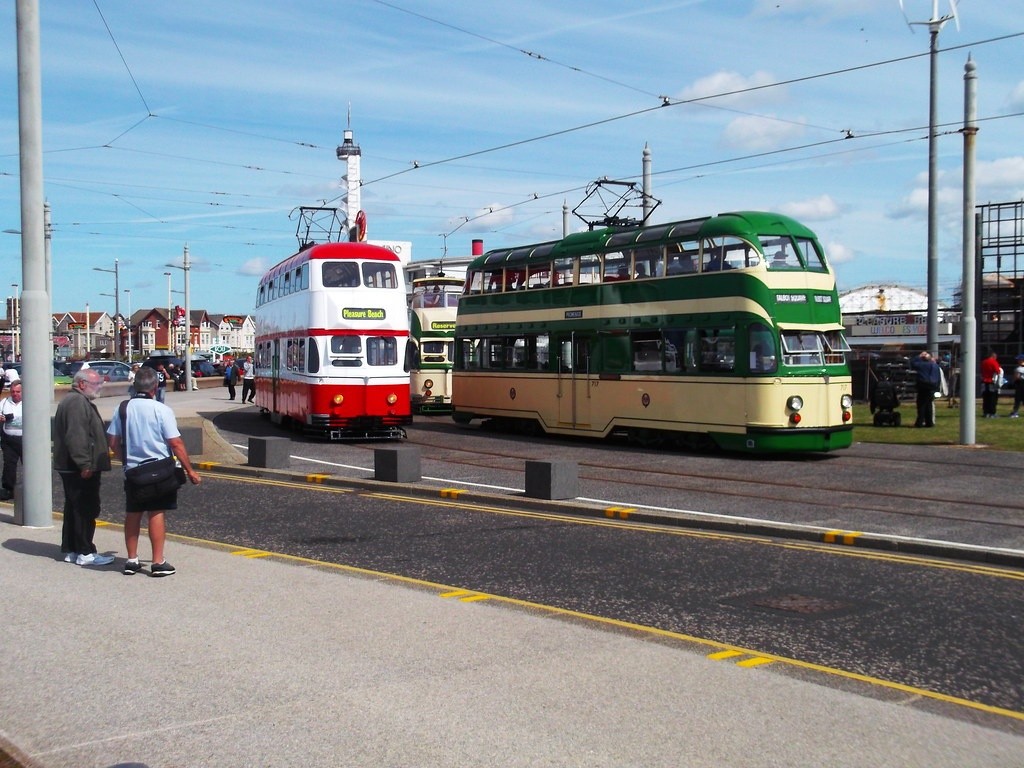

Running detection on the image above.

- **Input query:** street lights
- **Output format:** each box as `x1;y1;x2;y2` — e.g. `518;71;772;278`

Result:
13;283;20;358
2;193;53;311
163;241;192;391
93;257;133;365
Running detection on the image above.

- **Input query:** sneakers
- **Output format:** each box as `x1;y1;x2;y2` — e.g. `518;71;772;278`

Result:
64;552;77;562
124;562;142;575
76;553;114;566
151;561;176;577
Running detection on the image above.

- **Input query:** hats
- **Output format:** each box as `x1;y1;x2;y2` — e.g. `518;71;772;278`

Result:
1015;354;1024;360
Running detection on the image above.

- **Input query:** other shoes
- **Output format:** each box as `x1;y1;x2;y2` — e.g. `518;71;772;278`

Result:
248;399;254;404
985;414;989;417
1008;412;1019;418
990;415;998;419
0;488;14;500
193;387;198;389
242;401;246;404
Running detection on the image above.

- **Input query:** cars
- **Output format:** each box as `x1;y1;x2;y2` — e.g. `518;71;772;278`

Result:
75;355;255;382
4;361;69;376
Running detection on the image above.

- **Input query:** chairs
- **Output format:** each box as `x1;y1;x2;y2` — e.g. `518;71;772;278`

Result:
475;276;630;294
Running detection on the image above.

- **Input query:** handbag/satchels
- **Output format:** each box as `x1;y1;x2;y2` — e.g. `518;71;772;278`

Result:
223;378;228;387
126;456;181;500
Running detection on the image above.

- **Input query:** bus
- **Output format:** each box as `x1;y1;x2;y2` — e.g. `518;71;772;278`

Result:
408;262;467;415
254;205;411;443
451;181;856;458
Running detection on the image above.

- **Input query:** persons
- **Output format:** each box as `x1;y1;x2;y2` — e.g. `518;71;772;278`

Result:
635;263;649;279
0;379;22;500
911;352;940;427
770;251;789;266
53;369;115;566
5;364;20;383
980;353;1001;418
1009;354;1024;418
225;359;241;399
107;366;201;577
618;266;630;276
128;364;139;397
546;272;560;284
170;364;198;390
242;355;256;403
155;363;170;403
707;250;730;271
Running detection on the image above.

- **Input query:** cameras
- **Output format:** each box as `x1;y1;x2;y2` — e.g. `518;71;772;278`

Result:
4;413;14;420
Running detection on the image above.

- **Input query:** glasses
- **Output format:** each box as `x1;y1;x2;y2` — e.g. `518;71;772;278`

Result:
83;380;103;386
